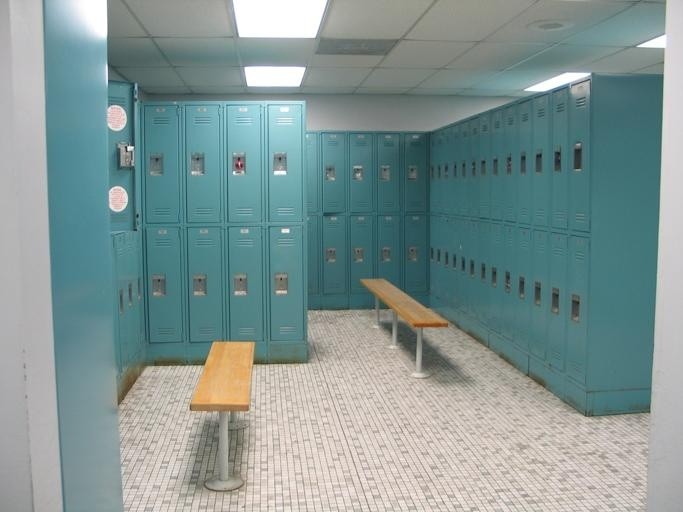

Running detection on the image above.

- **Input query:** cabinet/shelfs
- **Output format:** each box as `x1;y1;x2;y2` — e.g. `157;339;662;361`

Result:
427;73;663;419
107;79;138;405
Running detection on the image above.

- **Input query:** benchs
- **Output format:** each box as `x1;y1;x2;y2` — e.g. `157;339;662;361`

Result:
188;340;255;492
358;278;450;379
